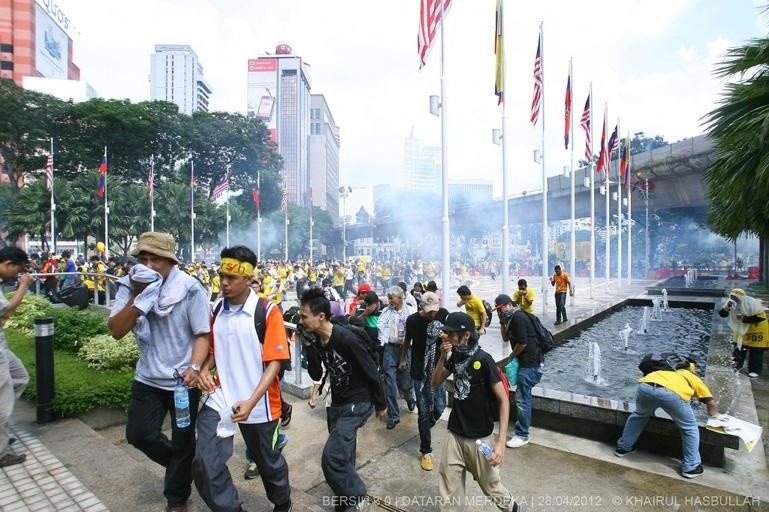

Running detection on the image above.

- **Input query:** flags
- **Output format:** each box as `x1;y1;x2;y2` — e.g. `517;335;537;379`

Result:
209;165;230;201
595;101;605;171
620;130;629;189
530;25;543;125
604;123;618;174
564;59;571;150
252;170;260;209
417;0;452;69
96;147;108;197
188;161;196;203
45;135;53;192
281;177;288;211
580;82;591;164
147;157;153;201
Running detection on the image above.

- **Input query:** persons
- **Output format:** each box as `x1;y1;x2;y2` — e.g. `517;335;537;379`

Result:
376;285;414;429
448;258;498;281
108;232;211;512
354;291;386;374
557;259;565;274
431;311;523;512
574;253;665;279
178;261;221;302
491;293;555;448
549;264;573;326
398;291;451;471
717;288;769;377
456;285;488;340
513;278;535;314
27;250;109;306
508;256;543;279
736;258;742;271
190;246;293;511
251;257;442;313
110;257;136;300
614;353;720;479
0;246;36;468
263;337;292;426
299;288;388;512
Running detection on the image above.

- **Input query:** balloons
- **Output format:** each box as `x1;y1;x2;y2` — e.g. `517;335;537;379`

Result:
97;241;105;251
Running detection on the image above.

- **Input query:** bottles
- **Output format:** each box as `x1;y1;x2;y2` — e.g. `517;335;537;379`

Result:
173;377;191;428
476;439;492;458
538;362;547;373
399;318;406;339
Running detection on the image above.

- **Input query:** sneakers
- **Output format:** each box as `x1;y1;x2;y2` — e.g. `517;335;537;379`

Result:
748;372;759;377
281;405;292;427
682;465;705;478
420;453;433;470
245;462;259;479
615;447;637;457
506;436;528;447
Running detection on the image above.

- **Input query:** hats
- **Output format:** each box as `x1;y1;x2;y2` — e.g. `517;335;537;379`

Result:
491;294;511;311
422;292;440;313
131;232;178;265
436;312;475;332
729;288;745;300
359;284;370;293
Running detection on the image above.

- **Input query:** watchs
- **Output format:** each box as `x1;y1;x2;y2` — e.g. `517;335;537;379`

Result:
189;364;201;374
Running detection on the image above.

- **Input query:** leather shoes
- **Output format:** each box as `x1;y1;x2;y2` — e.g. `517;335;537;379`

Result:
387;419;400;429
0;453;26;467
407;399;415;411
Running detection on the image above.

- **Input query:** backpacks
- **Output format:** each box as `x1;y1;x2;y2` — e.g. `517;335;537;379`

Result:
639;352;690;376
516;308;554;353
479;300;492;327
476;351;509;422
333;320;382;376
